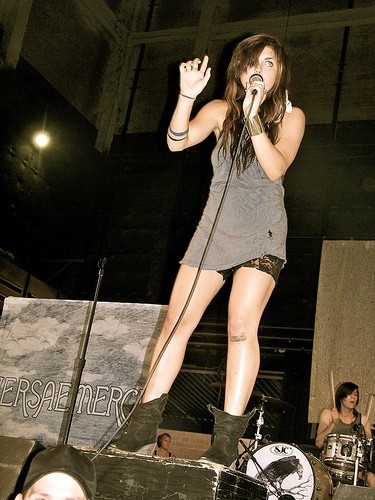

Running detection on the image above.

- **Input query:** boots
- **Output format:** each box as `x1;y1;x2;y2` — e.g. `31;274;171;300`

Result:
197;403;260;470
107;392;170;456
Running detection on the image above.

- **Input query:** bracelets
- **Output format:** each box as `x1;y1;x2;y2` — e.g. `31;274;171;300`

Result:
179;93;196;100
168;125;190;136
167;132;188;141
242;114;266;136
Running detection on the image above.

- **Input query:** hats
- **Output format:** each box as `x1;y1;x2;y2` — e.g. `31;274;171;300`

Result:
21;443;96;500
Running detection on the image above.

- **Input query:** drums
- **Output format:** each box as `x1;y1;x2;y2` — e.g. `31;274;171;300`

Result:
241;441;333;500
323;433;366;471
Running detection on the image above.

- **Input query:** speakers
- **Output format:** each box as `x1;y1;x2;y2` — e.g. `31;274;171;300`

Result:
0;436;46;500
20;446;269;500
331;483;375;500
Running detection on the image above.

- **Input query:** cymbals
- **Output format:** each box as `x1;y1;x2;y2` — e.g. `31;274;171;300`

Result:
252;392;286;404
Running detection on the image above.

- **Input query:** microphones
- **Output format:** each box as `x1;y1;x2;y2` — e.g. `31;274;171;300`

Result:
249;74;263;94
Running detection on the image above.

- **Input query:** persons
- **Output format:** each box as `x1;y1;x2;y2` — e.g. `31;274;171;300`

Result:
106;35;306;468
152;432;175;457
314;382;375;489
15;445;96;500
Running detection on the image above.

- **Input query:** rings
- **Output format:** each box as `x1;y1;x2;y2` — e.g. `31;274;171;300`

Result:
262;89;267;93
